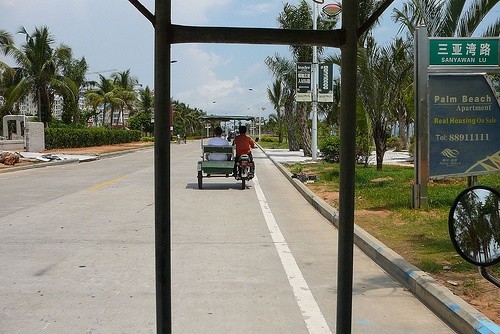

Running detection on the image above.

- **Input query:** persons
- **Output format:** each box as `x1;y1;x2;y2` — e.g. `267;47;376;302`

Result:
232;126;256;179
176;133;181;144
205;126;231;177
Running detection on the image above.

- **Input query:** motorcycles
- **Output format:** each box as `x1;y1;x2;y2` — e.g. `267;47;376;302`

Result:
197;113;259;190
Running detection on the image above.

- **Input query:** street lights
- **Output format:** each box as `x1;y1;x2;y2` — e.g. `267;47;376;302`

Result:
248;87;261;140
